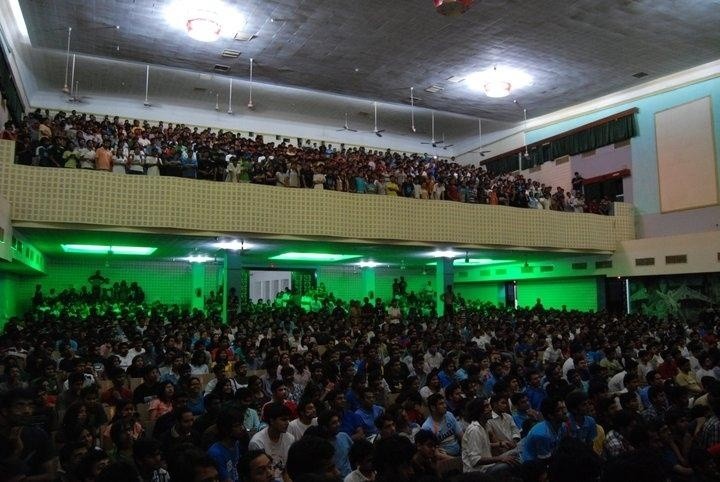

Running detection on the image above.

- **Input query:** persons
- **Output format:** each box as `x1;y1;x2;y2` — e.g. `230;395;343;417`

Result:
1;268;719;481
1;107;616;216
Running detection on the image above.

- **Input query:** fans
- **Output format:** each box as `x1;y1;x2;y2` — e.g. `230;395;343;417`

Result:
349;248;543;275
221;238;263;256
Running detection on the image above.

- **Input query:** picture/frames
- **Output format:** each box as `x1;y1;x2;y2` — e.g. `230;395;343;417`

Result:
653;93;719;215
